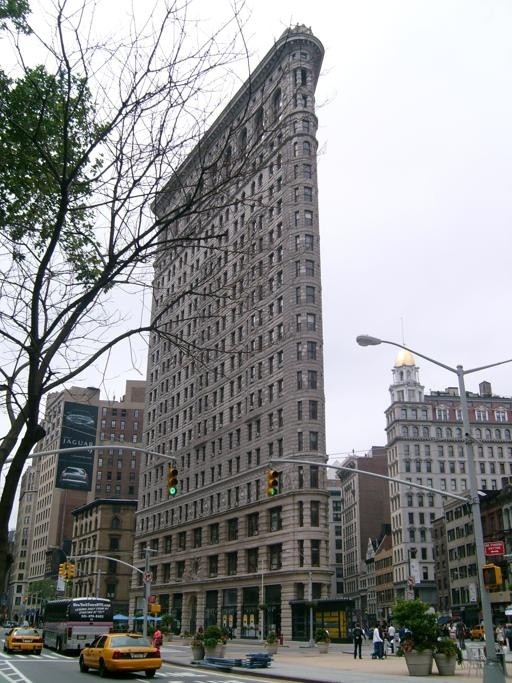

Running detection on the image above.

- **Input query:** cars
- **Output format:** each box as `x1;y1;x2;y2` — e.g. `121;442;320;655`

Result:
3;621;18;628
60;466;88;486
505;623;512;634
470;625;496;641
4;627;43;654
65;409;95;431
79;633;162;676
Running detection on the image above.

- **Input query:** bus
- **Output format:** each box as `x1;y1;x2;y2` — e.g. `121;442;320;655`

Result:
41;597;113;653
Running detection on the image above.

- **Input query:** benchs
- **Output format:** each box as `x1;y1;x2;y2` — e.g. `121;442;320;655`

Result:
466;643;508;677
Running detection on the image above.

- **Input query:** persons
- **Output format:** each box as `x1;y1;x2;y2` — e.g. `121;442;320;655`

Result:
152;624;163;651
198;624;204;634
348;614;512;661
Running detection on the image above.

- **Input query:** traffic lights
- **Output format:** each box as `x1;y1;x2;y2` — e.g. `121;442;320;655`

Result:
59;562;75;577
482;564;502;589
167;468;178;496
267;471;279;496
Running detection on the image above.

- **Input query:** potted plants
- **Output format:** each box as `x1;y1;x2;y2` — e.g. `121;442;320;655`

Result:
181;623;230;661
265;631;280;654
159;612;179;642
391;594;463;677
314;628;331;653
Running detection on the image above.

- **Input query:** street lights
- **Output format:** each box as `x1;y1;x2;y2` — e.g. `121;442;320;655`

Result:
356;335;512;683
